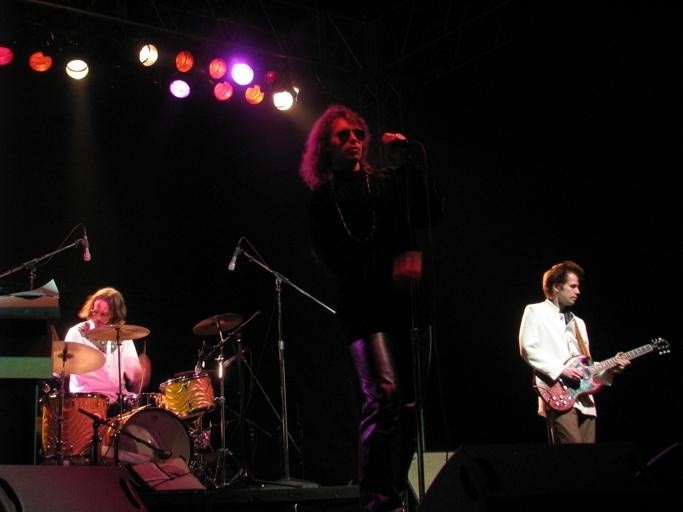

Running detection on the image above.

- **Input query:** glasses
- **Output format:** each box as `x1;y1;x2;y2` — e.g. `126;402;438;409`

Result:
336;129;366;145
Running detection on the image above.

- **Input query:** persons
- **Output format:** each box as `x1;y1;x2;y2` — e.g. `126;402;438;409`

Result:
298;104;425;511
63;286;143;419
517;259;633;444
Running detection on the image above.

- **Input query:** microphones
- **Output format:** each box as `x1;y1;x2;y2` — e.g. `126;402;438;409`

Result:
80;228;92;262
228;243;240;271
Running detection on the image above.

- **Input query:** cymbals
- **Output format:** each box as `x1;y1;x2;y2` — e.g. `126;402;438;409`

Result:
193;312;242;336
52;341;106;375
84;324;151;341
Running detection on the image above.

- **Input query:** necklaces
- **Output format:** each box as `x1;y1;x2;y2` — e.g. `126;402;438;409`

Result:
328;169;379;244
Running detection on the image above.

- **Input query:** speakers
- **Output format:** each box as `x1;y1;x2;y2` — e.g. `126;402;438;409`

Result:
0;464;148;512
137;484;362;512
416;444;605;512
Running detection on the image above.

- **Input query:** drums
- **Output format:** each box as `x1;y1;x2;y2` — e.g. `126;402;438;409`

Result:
160;374;215;417
99;406;193;467
129;392;160;409
40;392;109;457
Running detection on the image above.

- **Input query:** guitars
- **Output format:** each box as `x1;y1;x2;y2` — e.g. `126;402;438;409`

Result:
532;337;670;412
